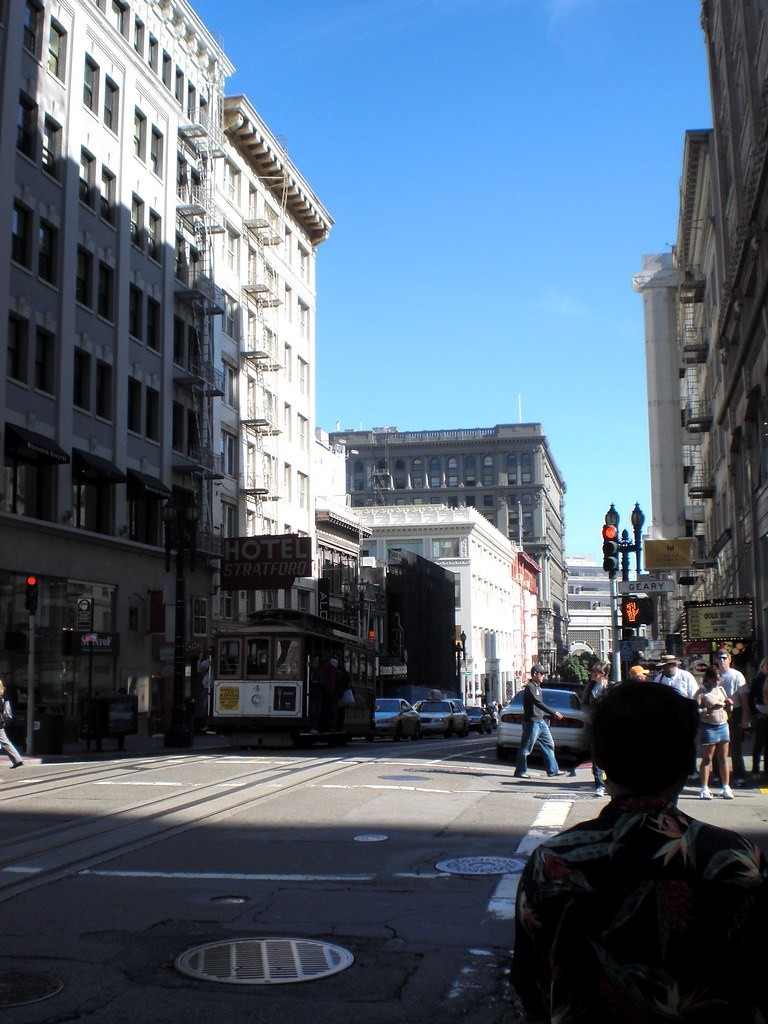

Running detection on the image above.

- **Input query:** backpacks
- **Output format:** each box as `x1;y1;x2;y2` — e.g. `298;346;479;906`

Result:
0;696;13;723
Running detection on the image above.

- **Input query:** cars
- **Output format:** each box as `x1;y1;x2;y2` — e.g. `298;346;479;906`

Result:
365;698;421;742
412;689;492;739
496;682;601;764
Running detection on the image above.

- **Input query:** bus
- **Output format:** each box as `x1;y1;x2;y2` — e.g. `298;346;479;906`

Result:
205;607;382;748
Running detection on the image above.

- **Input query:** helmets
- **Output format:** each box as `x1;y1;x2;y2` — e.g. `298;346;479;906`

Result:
629;665;650;679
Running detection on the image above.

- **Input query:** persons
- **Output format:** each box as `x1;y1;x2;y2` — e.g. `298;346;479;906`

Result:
717;650;750;787
0;681;23;768
310;654;341;733
630;666;649;680
583;663;610;796
748;657;768;780
693;667;733;799
511;682;768;1023
514;664;565;778
654;655;699;700
198;647;216;732
488;702;502;724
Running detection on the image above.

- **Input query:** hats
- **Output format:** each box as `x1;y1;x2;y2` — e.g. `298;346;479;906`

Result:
531;664;549;675
655;655;682;666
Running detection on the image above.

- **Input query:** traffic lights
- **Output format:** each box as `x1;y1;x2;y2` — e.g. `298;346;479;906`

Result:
622;597;656;627
24;576;38;611
602;525;619;573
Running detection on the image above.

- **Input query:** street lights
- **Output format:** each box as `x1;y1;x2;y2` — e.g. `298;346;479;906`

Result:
159;494;201;723
455;630;467;707
341;577;367;628
602;501;646;684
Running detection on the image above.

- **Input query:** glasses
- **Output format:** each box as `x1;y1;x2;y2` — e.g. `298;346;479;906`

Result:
718;657;729;660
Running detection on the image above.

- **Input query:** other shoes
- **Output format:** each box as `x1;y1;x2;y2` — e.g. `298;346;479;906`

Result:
595;786;606;797
734;777;747;787
548;771;565;777
711;774;721;786
699;788;713;800
10;761;23;768
723;789;734;799
514;772;530;778
752;771;761;782
